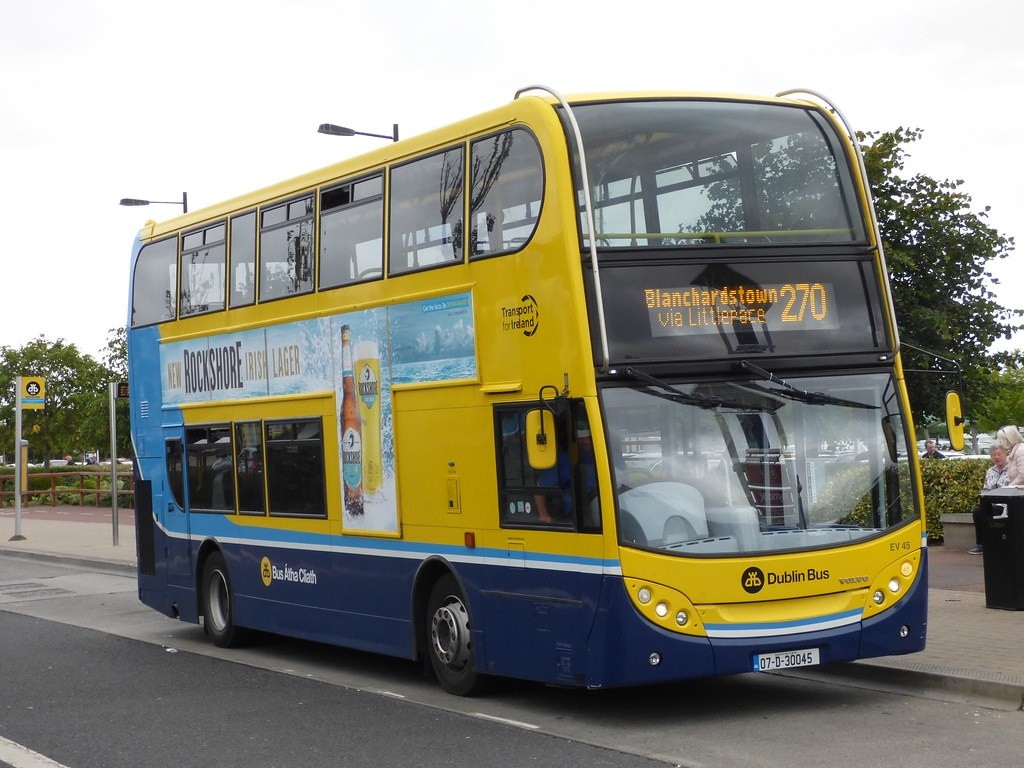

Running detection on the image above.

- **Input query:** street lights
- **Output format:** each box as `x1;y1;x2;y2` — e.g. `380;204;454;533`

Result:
121;191;187;213
316;122;399;143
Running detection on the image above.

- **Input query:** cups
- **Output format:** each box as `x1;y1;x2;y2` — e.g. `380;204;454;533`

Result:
353;341;384;504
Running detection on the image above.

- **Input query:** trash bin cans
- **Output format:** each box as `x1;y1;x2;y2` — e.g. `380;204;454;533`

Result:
977;487;1024;611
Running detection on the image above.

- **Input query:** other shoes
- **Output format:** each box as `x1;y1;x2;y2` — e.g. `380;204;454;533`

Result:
968;546;983;555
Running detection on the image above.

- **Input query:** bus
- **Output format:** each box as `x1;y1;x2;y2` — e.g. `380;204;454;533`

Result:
125;83;964;696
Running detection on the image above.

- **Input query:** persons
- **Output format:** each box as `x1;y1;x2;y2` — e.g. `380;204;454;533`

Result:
533;422;628;523
922;426;1024;555
65;454;100;466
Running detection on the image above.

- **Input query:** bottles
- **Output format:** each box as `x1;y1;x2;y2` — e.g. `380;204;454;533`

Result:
339;324;365;516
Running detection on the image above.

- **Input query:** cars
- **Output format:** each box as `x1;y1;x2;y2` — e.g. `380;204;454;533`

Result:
4;457;134;472
844;434;997;461
